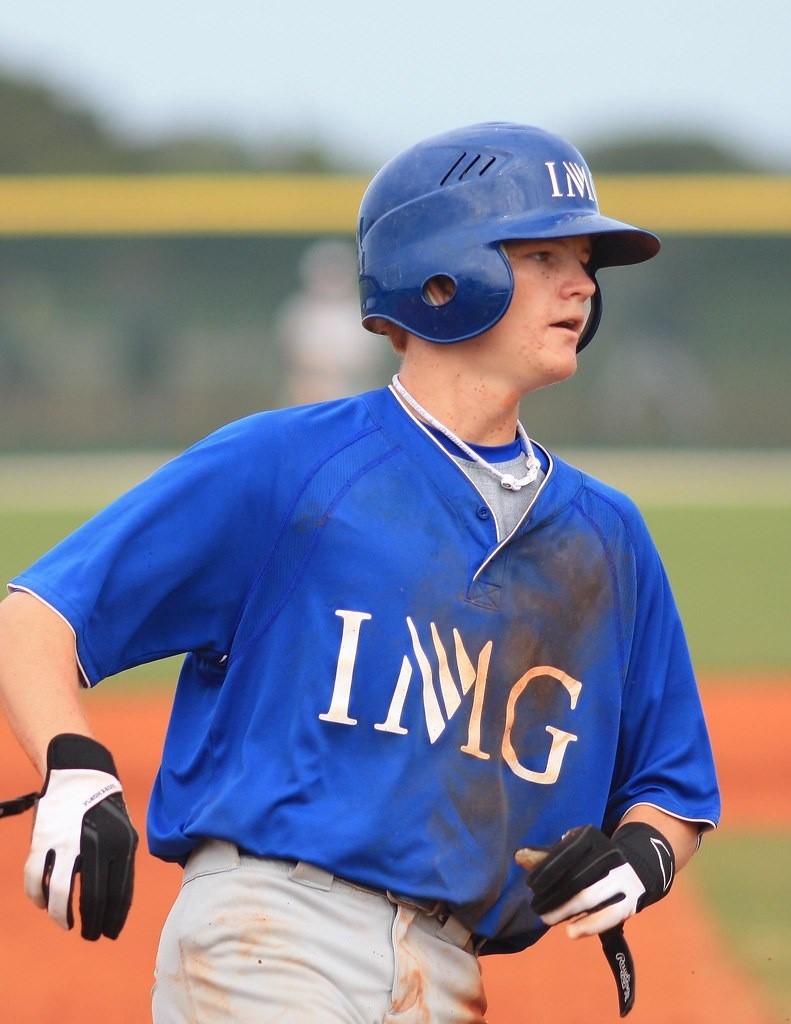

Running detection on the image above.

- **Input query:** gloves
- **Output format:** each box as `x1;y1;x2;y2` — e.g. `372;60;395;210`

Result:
514;815;676;1014
0;733;139;942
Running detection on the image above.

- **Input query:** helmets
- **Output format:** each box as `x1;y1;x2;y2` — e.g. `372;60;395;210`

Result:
354;125;660;354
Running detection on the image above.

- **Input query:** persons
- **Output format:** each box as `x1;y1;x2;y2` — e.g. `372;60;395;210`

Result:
0;122;722;1024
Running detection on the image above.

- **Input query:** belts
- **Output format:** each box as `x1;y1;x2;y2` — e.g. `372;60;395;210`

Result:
331;871;448;925
